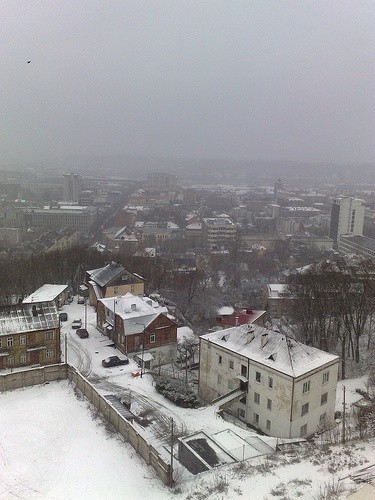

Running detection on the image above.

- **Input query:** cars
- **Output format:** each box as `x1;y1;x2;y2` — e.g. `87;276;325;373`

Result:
75;329;89;339
102;355;129;368
71;318;82;328
78;296;84;303
